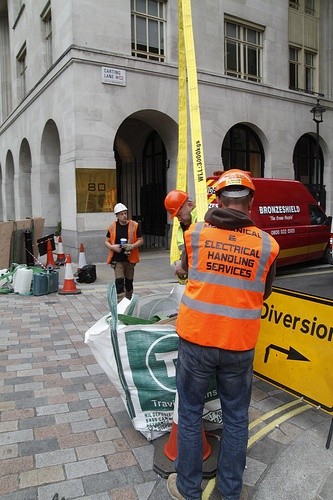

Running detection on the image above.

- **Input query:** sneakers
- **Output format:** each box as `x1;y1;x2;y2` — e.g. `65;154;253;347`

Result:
167;472;188;500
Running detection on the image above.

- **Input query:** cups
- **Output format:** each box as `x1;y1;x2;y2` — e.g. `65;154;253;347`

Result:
120;238;127;248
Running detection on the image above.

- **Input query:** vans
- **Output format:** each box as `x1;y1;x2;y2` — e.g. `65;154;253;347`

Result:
206;170;333;268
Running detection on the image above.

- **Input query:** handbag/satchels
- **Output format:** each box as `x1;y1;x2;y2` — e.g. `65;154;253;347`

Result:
76;264;96;284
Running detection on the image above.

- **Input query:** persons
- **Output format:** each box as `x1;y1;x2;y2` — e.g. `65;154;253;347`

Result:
165;168;280;500
164;189;207;286
104;203;143;304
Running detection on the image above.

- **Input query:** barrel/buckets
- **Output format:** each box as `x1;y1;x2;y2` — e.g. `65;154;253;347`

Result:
14;267;33;293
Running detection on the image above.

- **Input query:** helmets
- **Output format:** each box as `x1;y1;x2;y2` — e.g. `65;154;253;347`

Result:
164;189;188;219
114;203;128;214
215;169;255;195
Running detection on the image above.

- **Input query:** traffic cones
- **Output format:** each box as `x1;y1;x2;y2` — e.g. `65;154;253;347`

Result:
44;237;60;268
58;254;82;295
73;243;88;277
54;236;67;267
